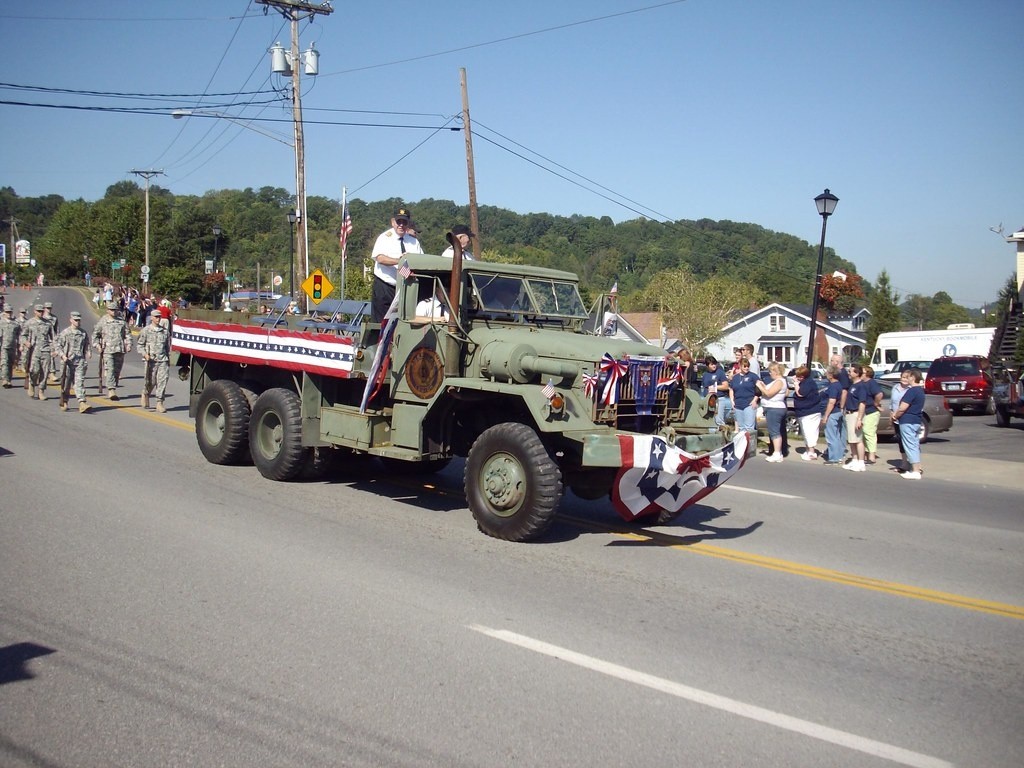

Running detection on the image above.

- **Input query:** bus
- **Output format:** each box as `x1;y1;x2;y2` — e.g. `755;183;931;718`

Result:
869;322;997;371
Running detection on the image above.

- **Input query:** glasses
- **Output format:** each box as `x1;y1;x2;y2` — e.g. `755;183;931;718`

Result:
743;349;748;351
742;365;749;368
395;219;408;226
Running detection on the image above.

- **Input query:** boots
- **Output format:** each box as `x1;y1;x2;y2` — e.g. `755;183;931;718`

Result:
61;402;69;410
109;389;117;398
28;385;35;396
50;373;59;381
79;402;91;413
156;402;166;413
39;389;47;400
141;395;149;407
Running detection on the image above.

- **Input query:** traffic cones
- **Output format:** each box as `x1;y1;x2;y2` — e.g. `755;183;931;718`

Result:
2;283;31;292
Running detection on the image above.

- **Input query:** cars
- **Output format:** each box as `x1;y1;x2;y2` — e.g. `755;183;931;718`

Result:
785;378;952;444
777;360;861;380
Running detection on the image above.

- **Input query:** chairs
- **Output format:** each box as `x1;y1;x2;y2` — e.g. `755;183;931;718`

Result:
296;300;370;336
252;296;294;329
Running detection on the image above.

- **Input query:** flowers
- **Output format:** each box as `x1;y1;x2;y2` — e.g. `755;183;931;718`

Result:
820;271;863;306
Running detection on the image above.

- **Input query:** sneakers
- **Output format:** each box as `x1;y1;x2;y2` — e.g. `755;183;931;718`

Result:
767;452;784;462
902;471;921;480
842;459;866;472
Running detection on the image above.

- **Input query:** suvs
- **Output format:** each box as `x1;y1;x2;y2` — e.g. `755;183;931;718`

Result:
992;362;1024;427
924;353;996;416
879;359;934;389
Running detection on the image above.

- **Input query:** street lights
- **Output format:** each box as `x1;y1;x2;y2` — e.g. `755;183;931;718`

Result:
286;207;297;301
805;188;840;369
170;108;310;316
212;224;222;310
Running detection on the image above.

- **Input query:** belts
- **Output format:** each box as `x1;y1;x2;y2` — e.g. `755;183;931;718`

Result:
846;410;856;414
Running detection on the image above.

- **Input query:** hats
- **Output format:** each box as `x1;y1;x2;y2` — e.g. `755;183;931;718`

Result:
151;310;161;317
393;208;411;222
453;224;476;237
3;301;82;321
107;303;119;309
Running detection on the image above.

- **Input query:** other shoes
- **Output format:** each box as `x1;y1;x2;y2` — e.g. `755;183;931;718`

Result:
3;380;12;388
801;451;817;461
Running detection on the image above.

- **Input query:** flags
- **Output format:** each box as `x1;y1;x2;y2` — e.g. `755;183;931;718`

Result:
608;282;618;299
340;195;353;260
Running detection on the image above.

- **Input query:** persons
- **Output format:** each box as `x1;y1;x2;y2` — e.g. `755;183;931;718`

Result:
178;294;188;308
729;343;761;380
223;301;233;311
36;272;44;287
157;294;173;330
232;304;238;311
793;366;821;461
442;225;477;261
92;288;100;310
102;279;139;328
85;271;91;287
91;302;133;401
411;275;449;326
406;221;422;237
671;348;701;395
366;209;425;349
0;294;59;401
888;366;925;480
136;310;171;413
261;304;269;314
134;293;159;328
755;361;789;463
729;358;761;456
822;354;884;472
0;272;8;288
477;282;529;323
55;311;92;413
701;356;728;427
9;271;15;289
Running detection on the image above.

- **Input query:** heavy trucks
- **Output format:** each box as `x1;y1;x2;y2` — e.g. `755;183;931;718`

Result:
171;232;759;543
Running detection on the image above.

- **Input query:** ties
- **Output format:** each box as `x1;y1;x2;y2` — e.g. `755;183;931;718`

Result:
399;237;406;253
463;252;466;260
505;306;511;317
440;304;445;316
